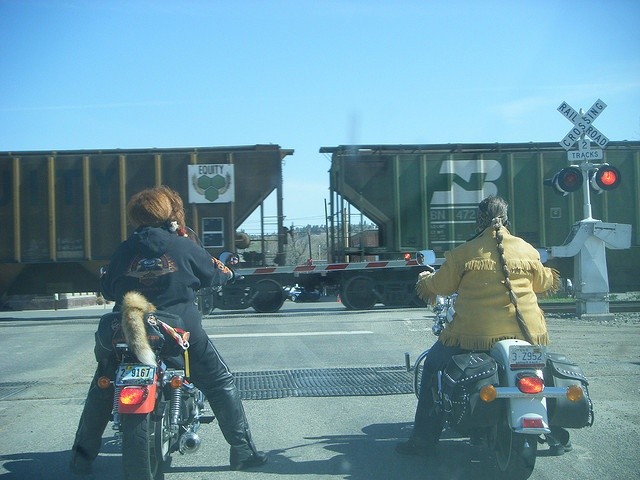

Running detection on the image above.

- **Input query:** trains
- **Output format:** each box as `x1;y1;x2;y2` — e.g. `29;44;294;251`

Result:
0;138;640;314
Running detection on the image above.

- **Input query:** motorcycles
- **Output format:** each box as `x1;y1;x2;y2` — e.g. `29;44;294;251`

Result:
405;249;584;480
101;226;245;480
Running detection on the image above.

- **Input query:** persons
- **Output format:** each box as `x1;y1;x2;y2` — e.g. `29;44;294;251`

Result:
68;187;268;476
396;196;574;458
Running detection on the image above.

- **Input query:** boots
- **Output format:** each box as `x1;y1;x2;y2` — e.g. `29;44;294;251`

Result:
68;402;113;478
207;387;268;469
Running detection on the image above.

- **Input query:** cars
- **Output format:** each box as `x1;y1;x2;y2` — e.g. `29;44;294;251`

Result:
287;286;322;301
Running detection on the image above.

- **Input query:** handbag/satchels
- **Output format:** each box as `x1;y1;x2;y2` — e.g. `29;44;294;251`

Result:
544;352;594;429
437;351;499;433
94;310;188;371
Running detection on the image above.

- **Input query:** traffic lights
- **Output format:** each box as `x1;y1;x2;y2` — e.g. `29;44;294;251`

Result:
595;165;621;191
557;166;584;192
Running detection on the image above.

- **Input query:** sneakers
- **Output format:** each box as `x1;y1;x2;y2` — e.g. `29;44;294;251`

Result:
395;434;440;456
549;439;572;456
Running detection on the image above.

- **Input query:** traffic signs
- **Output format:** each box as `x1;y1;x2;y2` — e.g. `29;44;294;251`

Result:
567;149;603;162
